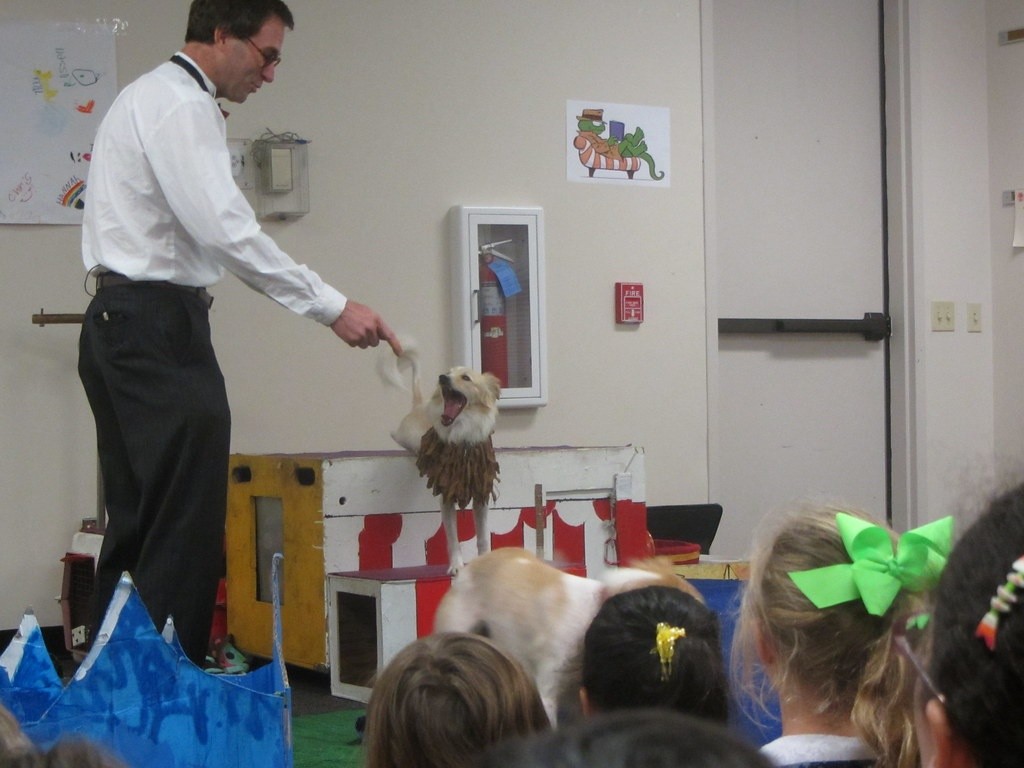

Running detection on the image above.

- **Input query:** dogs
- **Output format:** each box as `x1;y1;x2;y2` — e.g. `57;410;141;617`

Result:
429;547;707;731
390;348;501;575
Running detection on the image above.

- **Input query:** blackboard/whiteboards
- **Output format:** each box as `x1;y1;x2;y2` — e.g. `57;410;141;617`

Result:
704;2;893;341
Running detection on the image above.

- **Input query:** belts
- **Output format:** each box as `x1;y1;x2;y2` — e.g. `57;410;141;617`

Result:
97;275;214;310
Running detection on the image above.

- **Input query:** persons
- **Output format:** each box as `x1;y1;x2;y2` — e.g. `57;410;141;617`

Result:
578;584;729;728
362;630;554;768
0;701;128;768
730;503;954;768
914;482;1024;768
475;710;776;768
78;1;403;670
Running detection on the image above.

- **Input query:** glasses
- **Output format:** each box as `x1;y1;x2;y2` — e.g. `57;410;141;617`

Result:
242;33;281;68
892;609;960;733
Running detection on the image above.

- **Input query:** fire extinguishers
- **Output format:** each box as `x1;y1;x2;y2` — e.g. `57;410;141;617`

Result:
476;238;515;389
